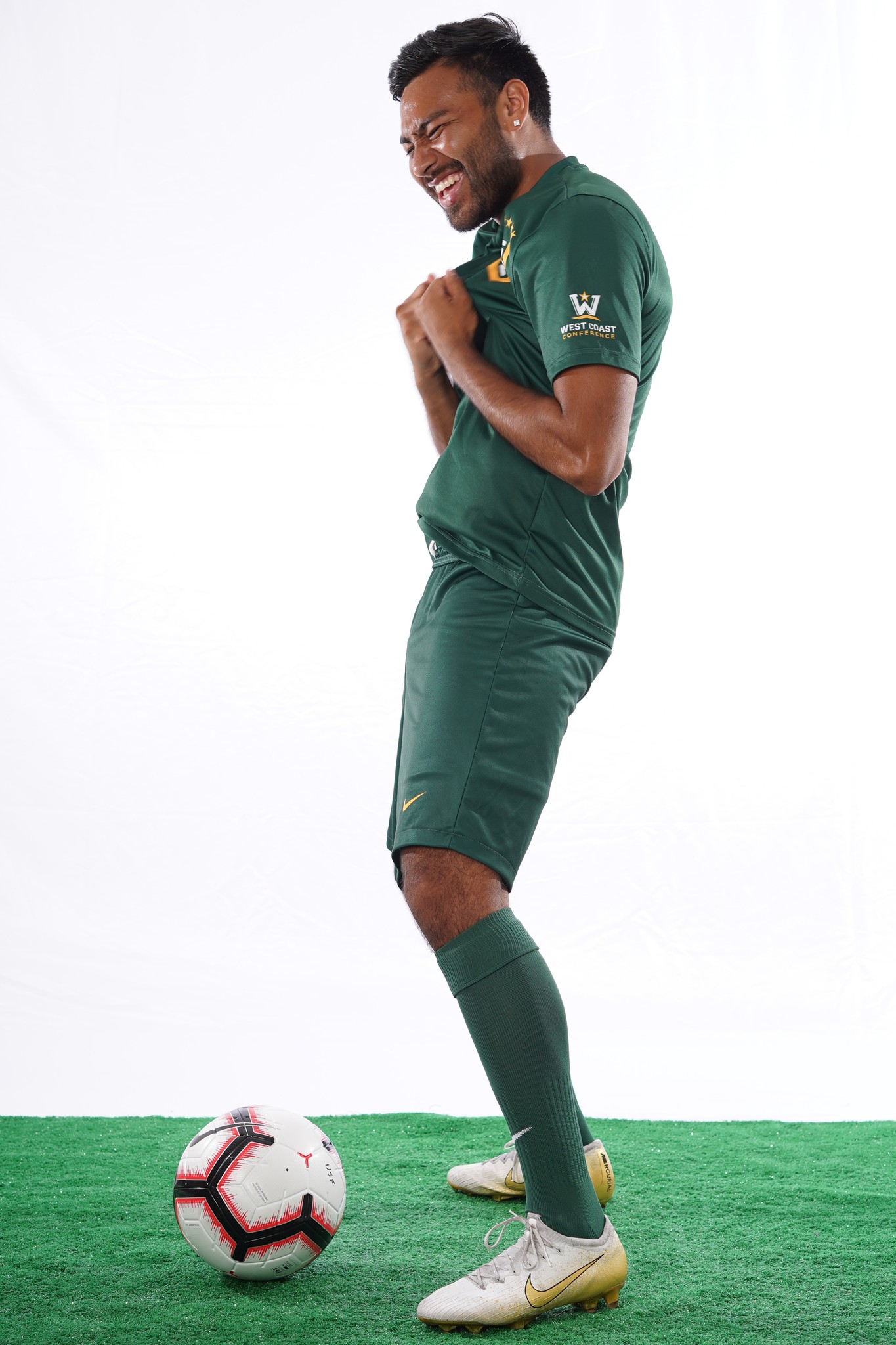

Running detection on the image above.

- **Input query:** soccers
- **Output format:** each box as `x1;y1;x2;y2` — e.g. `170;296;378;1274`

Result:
171;1104;348;1282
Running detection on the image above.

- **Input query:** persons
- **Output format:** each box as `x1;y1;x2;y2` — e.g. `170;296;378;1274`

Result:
381;14;674;1326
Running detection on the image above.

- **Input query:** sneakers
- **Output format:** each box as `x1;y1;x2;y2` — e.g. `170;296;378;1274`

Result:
446;1139;615;1208
414;1210;628;1333
